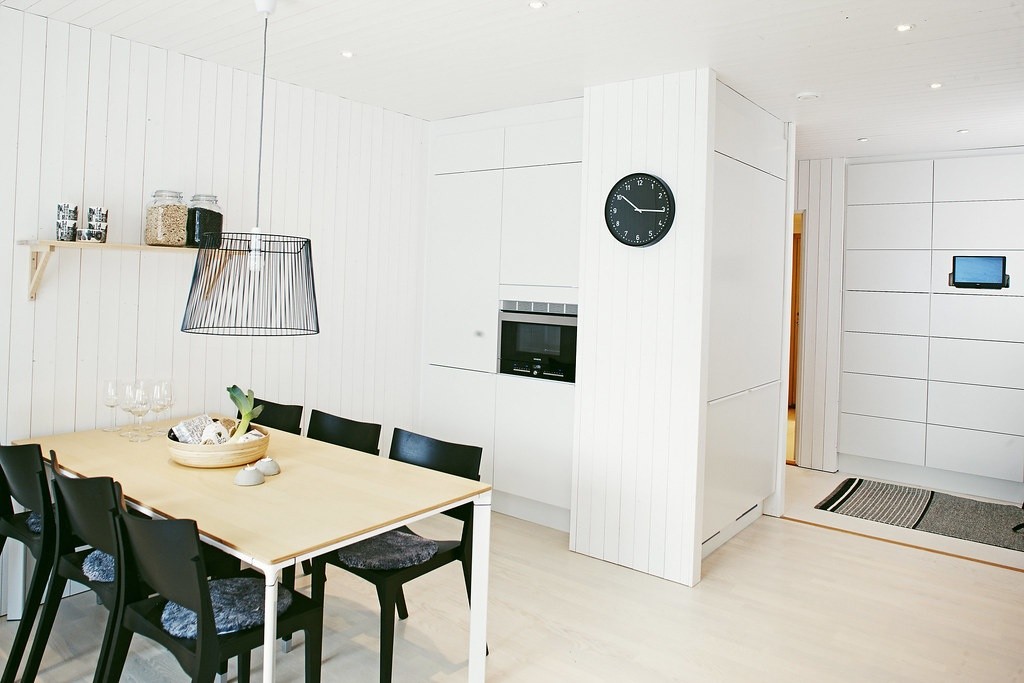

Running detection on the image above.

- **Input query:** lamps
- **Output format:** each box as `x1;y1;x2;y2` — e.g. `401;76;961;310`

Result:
179;1;320;337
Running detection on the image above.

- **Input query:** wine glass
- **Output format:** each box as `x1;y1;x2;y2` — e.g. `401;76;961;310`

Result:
101;376;175;442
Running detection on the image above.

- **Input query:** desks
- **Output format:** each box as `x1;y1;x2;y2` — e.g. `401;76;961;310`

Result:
12;414;490;683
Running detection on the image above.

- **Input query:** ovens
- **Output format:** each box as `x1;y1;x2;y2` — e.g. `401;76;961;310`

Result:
497;300;578;383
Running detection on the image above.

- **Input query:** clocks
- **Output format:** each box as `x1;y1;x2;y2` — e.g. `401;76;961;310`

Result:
604;172;675;247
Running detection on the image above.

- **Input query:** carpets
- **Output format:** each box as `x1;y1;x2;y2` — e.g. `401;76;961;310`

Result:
814;477;1024;551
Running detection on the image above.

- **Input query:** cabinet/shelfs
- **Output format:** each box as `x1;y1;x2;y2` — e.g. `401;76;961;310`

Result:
415;65;795;587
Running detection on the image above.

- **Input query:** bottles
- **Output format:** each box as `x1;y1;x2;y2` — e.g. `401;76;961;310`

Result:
145;190;188;247
186;194;223;249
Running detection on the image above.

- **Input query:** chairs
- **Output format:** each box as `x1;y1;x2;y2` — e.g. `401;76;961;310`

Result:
0;398;483;683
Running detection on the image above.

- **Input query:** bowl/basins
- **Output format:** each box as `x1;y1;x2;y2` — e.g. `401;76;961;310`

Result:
234;466;265;486
253;458;281;476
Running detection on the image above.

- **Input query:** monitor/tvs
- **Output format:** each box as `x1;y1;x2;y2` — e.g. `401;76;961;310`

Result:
954;256;1007;289
